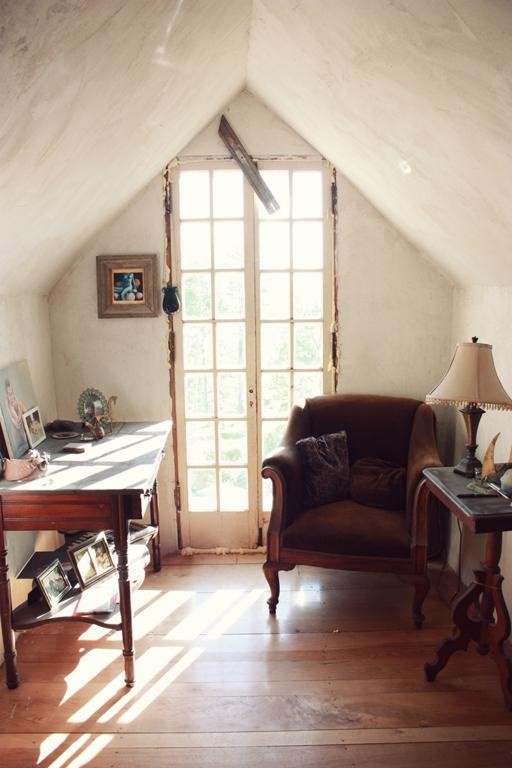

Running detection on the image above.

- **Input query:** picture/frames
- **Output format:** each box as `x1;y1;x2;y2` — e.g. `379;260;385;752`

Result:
22;406;47;450
78;388;109;425
35;559;73;610
96;255;161;317
104;521;158;552
66;530;118;591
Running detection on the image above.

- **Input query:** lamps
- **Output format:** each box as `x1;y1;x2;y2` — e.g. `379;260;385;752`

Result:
424;337;512;479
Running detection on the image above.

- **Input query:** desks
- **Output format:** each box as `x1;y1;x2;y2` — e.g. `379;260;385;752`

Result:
421;466;512;705
0;420;174;689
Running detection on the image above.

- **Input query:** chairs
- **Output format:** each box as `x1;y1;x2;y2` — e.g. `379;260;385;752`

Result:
260;393;443;621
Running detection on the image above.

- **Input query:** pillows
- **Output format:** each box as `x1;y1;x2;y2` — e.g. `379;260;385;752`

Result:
350;456;406;508
296;429;350;508
